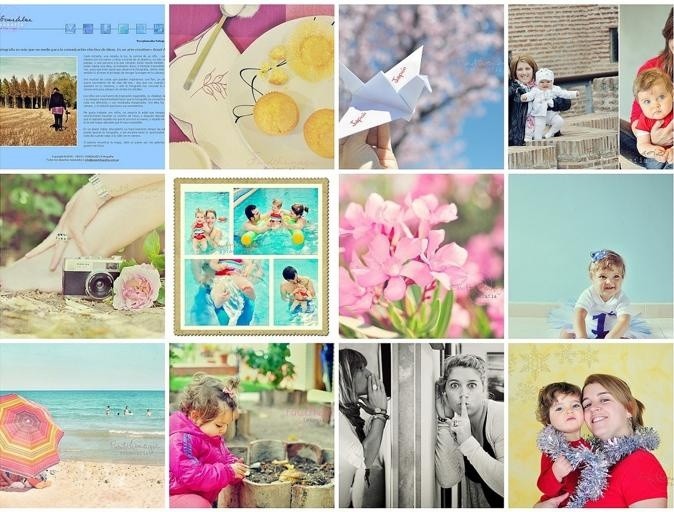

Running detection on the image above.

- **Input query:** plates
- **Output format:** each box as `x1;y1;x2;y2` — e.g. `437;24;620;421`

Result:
228;15;335;169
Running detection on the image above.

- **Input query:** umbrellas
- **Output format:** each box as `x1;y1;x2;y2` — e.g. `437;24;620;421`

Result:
0;393;65;481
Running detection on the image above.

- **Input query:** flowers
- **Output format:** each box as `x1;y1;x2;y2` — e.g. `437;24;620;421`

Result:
113;231;165;311
338;192;468;338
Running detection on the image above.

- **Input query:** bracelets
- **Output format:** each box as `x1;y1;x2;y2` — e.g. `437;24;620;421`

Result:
370;413;389;423
438;416;450;427
89;175;114;204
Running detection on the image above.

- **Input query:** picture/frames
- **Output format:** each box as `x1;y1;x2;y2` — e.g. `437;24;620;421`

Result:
173;178;328;336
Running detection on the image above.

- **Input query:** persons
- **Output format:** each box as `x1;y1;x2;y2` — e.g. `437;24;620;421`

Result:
507;56;580;144
536;384;592;504
556;247;633;340
47;87;70;133
1;470;50;489
338;348;391;506
630;7;674;169
169;371;251;508
1;174;165;295
339;107;398;169
103;401;154;419
187;197;317;326
434;354;504;507
533;370;669;507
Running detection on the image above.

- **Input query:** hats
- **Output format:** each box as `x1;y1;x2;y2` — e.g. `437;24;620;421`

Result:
536;68;554;83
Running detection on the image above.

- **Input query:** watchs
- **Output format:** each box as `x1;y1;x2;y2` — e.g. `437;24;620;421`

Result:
373;407;387;413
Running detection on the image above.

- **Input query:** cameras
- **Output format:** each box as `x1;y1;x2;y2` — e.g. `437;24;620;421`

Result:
62;255;127;303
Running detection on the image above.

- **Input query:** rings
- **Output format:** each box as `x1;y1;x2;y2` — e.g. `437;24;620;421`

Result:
55;231;72;244
453;420;459;428
372;383;378;392
